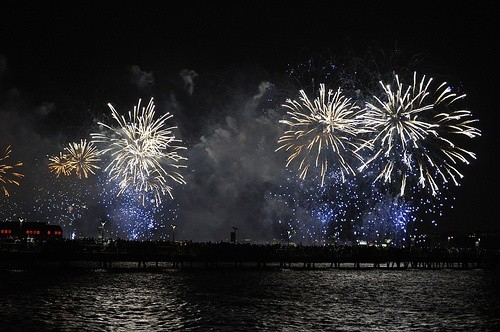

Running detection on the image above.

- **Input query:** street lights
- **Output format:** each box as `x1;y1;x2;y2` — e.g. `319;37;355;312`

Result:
19;217;24;245
101;221;107;247
171;225;176;242
